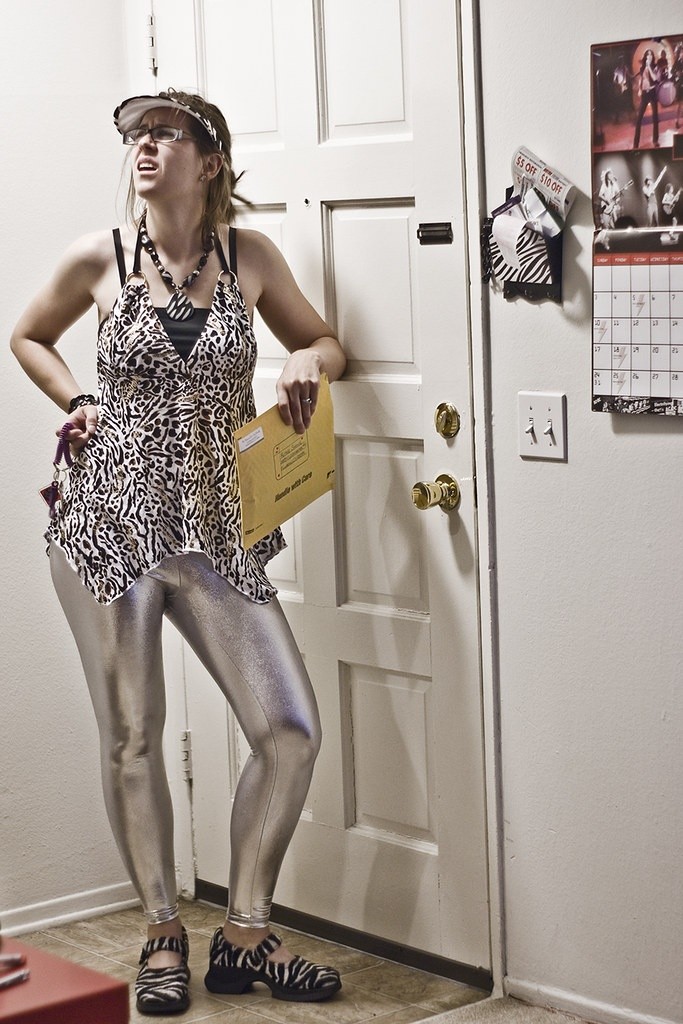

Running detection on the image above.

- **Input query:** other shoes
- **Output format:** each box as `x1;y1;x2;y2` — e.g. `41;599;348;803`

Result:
135;926;190;1014
205;926;342;1001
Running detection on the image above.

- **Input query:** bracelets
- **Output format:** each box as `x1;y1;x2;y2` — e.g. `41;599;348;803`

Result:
68;394;98;415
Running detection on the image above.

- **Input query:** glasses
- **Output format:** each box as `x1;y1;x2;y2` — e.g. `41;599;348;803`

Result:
123;126;192;142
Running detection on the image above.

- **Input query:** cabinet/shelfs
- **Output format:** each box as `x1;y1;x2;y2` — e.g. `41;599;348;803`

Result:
0;937;130;1024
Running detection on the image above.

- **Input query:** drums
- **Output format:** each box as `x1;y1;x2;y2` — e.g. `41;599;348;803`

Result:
656;79;677;106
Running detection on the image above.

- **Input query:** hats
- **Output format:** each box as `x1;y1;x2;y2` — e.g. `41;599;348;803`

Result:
114;96;222;151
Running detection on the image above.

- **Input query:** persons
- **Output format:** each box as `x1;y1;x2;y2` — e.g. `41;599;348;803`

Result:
597;49;683;242
9;88;347;1018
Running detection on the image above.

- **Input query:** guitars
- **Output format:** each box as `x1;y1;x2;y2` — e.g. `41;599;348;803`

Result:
662;186;682;215
600;180;634;215
612;72;641;94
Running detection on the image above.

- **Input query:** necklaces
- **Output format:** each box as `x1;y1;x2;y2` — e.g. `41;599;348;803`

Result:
140;208;215;321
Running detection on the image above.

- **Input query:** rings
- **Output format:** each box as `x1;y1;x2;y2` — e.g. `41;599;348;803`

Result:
302;398;311;404
